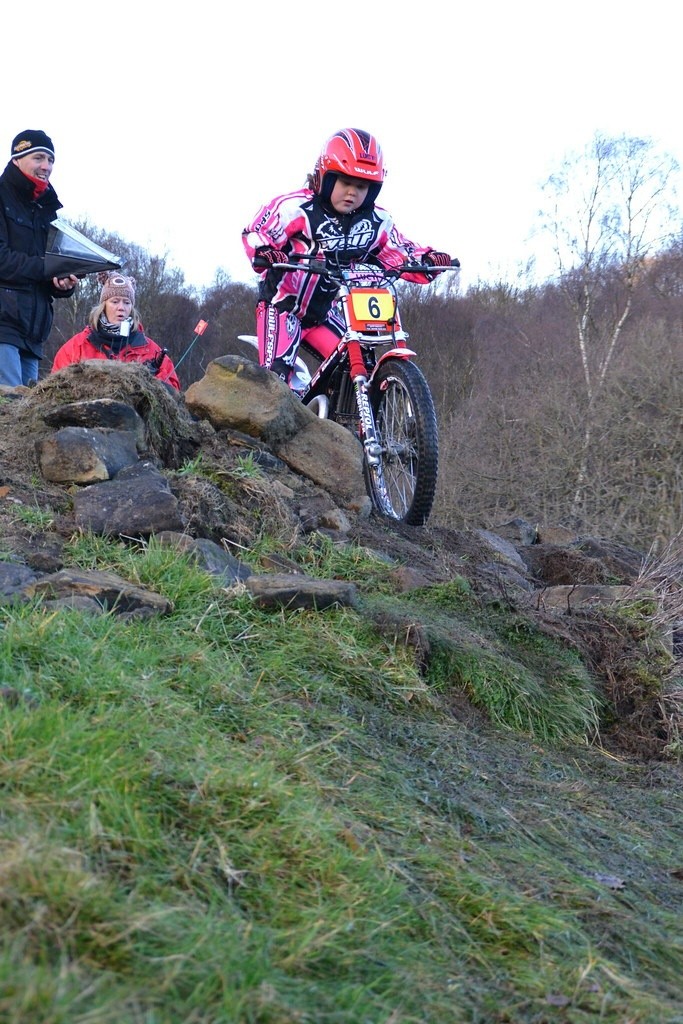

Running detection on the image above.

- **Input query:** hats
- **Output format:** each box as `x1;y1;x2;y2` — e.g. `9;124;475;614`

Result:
96;271;137;306
11;129;55;164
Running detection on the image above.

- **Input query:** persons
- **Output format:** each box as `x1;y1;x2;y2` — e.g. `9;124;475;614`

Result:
241;128;452;386
0;130;78;389
51;271;180;397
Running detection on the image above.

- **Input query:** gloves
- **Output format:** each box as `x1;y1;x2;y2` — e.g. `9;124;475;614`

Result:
256;247;289;269
421;252;452;281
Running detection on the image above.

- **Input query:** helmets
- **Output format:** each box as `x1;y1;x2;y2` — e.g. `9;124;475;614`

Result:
309;127;384;206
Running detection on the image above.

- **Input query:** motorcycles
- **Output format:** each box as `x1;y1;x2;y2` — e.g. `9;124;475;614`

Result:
250;250;464;526
